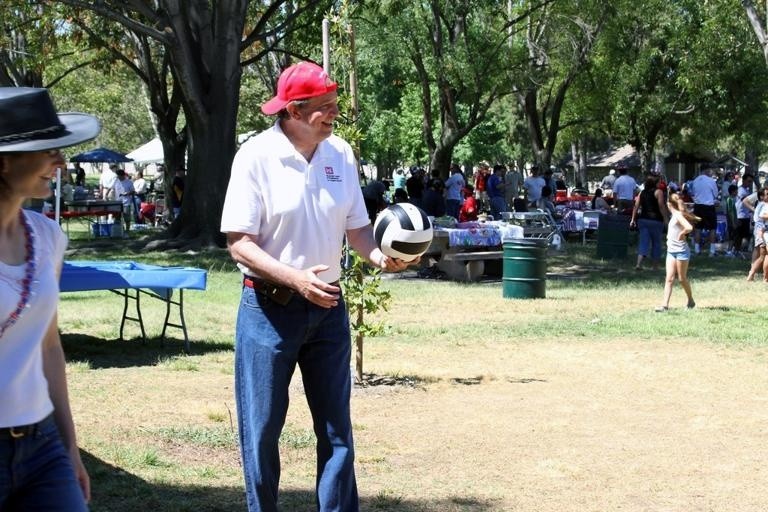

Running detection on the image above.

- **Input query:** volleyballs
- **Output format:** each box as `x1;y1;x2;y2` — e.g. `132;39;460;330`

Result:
373;203;433;263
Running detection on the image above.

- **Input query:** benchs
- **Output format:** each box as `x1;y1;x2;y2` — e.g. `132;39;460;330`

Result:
449;250;504;283
523;228;551;234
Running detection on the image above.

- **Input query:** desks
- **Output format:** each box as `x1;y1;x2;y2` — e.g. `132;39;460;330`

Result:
556;206;618;246
59;260;207;353
419;219;524;274
45;211;124;244
500;211;547;225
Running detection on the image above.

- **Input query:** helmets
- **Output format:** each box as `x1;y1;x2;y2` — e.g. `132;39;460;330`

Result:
410;165;420;174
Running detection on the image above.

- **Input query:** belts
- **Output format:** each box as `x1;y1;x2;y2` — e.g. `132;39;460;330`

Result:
0;418;49;439
244;277;340;297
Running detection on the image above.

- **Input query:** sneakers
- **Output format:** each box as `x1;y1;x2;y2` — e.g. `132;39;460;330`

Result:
655;305;668;312
687;299;695;309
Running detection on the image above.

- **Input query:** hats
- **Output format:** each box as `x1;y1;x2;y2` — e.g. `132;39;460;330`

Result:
259;62;339;117
0;86;101;154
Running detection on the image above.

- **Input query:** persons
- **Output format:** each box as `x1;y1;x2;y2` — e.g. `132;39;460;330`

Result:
220;59;420;511
0;86;101;511
588;166;718;270
362;157;523;222
655;194;701;312
59;161;148;232
523;167;558;208
723;173;767;281
570;181;588;198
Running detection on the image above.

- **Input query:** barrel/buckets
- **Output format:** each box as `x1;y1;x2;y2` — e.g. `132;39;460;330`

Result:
500;236;550;299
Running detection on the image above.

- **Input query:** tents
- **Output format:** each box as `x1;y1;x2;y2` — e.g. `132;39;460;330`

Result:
124;137;188;177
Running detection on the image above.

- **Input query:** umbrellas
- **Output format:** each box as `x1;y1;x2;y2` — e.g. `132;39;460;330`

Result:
69;148;134;163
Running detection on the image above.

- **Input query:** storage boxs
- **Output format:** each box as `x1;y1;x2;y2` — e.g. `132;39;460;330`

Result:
64;199;124;213
91;223;114;236
